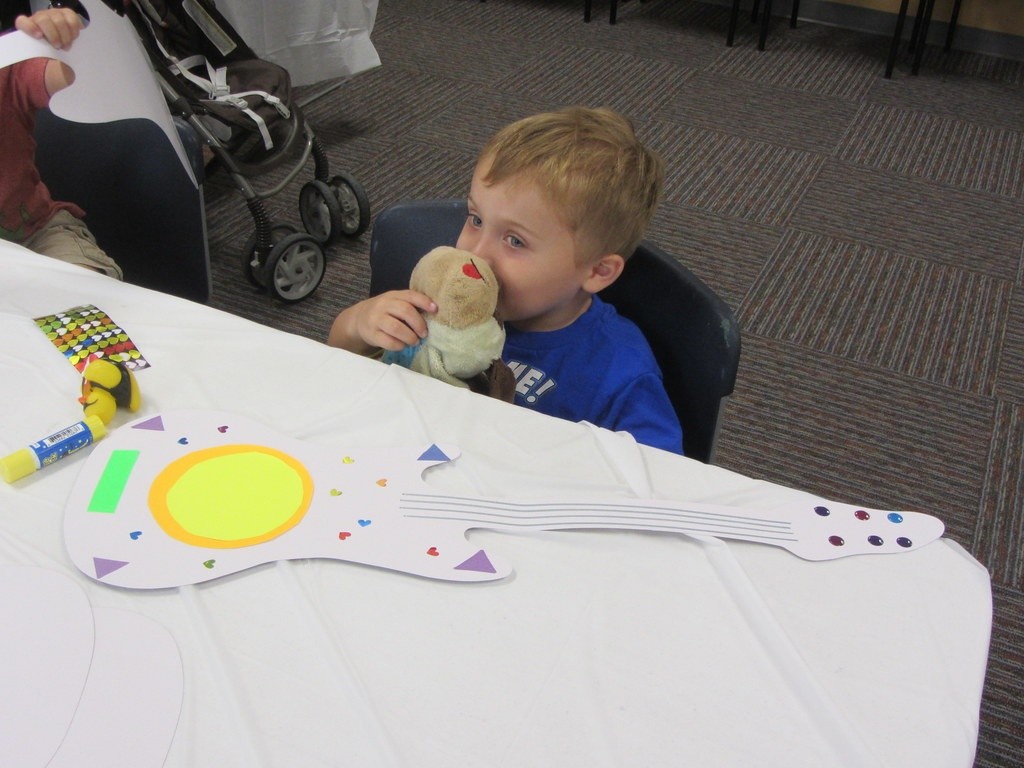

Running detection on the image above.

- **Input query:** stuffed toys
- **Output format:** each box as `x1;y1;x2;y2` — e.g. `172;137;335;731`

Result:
408;245;516;405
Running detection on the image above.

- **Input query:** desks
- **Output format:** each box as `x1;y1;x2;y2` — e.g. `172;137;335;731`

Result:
1;238;994;768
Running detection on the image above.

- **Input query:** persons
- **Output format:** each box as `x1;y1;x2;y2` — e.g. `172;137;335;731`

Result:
0;7;124;281
323;102;684;453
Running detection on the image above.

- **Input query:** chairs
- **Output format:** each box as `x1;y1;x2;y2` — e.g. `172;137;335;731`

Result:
18;109;210;305
371;200;741;468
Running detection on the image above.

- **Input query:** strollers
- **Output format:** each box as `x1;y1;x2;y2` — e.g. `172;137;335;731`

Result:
32;0;374;305
